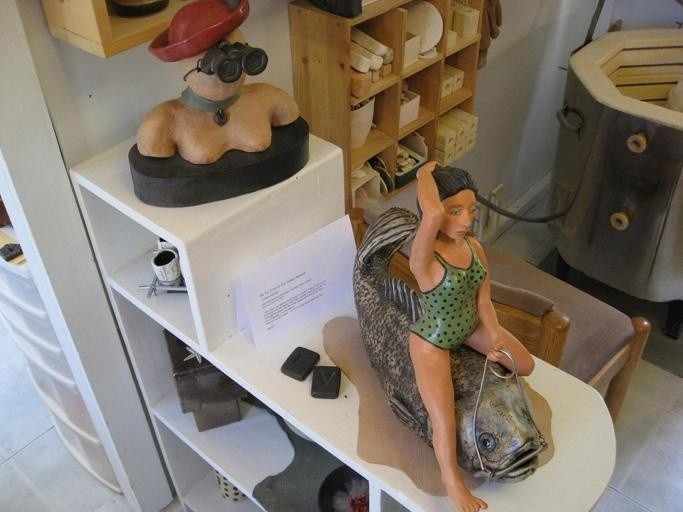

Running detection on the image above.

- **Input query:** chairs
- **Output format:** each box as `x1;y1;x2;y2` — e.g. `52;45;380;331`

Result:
350;207;650;421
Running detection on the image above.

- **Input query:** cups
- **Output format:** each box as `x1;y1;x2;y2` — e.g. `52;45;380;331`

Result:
151;249;182;286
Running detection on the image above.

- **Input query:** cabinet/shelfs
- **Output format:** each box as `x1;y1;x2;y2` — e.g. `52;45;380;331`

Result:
1;1;172;511
40;0;194;59
68;134;619;512
288;2;484;210
